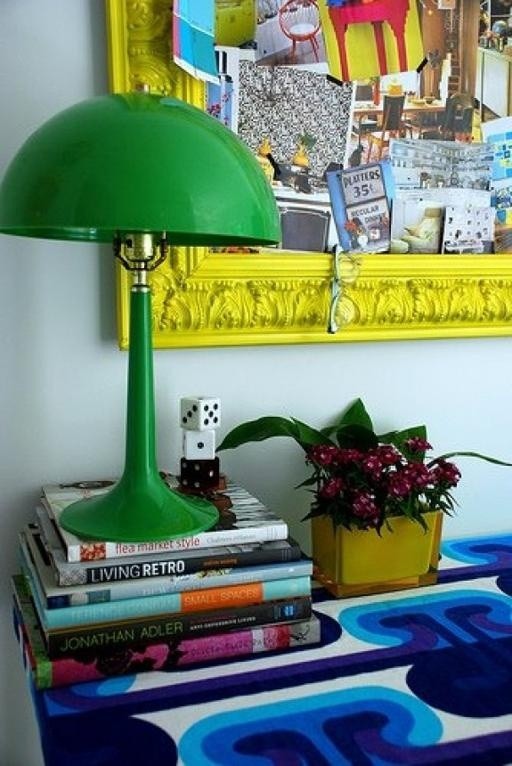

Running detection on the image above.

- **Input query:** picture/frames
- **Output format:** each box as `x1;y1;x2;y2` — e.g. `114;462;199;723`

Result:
103;1;510;352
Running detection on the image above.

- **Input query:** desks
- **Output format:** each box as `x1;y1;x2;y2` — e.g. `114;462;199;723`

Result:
9;534;512;766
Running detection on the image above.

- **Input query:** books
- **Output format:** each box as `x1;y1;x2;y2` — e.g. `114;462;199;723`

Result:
7;470;323;694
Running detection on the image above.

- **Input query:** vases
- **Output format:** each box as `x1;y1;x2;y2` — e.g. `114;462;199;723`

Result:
304;508;442;599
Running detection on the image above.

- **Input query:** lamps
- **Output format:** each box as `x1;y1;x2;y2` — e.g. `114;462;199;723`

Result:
2;90;283;536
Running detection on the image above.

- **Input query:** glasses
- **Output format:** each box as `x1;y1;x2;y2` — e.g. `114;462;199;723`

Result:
327;243;361;333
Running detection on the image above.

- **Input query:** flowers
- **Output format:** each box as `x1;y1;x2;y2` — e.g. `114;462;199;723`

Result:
217;398;512;535
